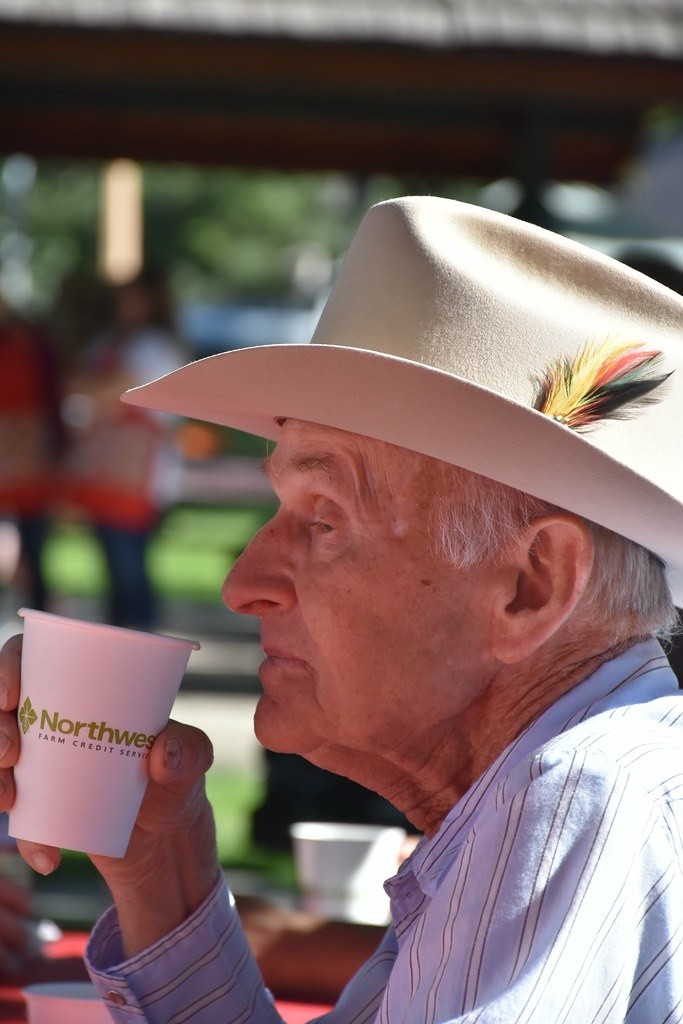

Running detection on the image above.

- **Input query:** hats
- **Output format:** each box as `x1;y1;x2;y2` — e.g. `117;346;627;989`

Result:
119;196;682;611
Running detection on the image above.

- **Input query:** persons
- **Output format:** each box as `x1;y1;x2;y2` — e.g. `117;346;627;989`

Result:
0;195;683;1024
0;274;188;631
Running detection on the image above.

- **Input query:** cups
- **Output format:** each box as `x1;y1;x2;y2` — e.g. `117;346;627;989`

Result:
288;820;407;926
9;607;198;859
